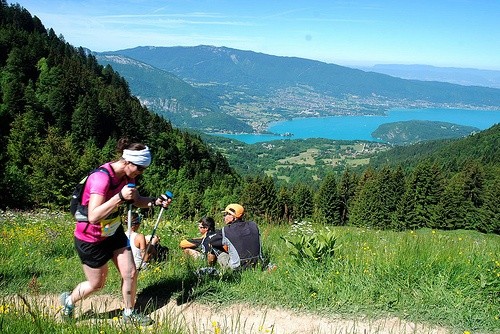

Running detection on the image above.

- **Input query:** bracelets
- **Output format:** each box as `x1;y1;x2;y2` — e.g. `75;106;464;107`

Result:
119;191;125;202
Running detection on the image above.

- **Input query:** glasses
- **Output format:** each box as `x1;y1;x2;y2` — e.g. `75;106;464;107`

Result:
127;162;146;172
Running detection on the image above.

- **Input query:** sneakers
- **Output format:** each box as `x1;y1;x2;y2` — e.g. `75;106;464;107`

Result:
60;293;74;321
123;312;152;326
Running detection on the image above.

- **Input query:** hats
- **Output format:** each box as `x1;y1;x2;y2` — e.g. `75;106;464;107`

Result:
221;204;244;217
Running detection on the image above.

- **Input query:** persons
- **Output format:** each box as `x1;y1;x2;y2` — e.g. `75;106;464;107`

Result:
206;203;265;274
123;211;169;272
59;137;171;327
179;217;223;262
